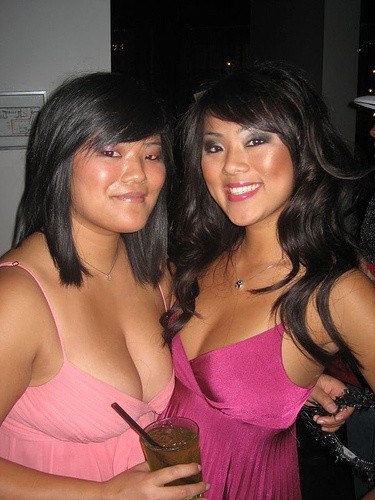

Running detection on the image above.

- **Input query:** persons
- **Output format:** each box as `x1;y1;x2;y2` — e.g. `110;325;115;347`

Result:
160;64;375;500
0;73;355;500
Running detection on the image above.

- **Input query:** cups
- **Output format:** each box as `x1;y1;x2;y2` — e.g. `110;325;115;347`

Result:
141;417;203;500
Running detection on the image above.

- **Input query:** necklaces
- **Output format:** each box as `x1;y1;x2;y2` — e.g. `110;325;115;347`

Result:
78;252;119;281
235;245;289;289
